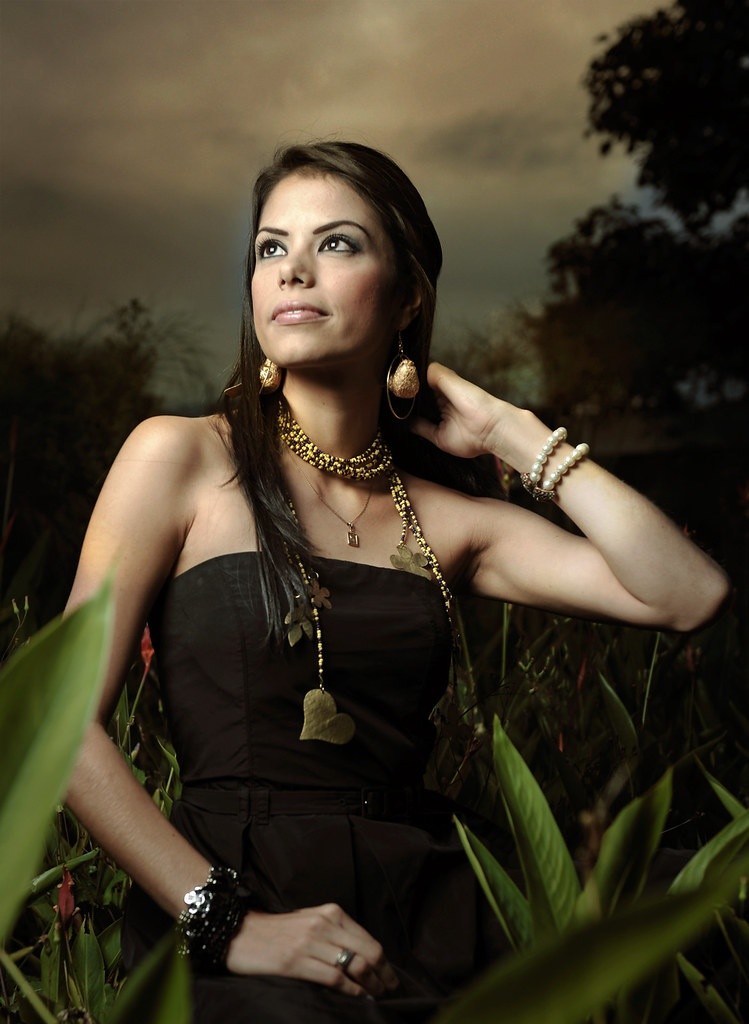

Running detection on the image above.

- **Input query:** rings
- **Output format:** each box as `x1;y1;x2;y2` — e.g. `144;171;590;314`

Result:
334;947;354;973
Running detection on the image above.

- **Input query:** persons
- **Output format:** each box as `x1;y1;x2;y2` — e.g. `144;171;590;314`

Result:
60;139;732;1024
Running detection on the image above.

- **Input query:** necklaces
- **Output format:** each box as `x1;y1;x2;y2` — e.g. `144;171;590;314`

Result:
262;394;476;749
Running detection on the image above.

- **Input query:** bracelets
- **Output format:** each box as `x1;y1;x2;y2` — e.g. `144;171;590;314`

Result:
520;426;591;503
174;864;255;974
